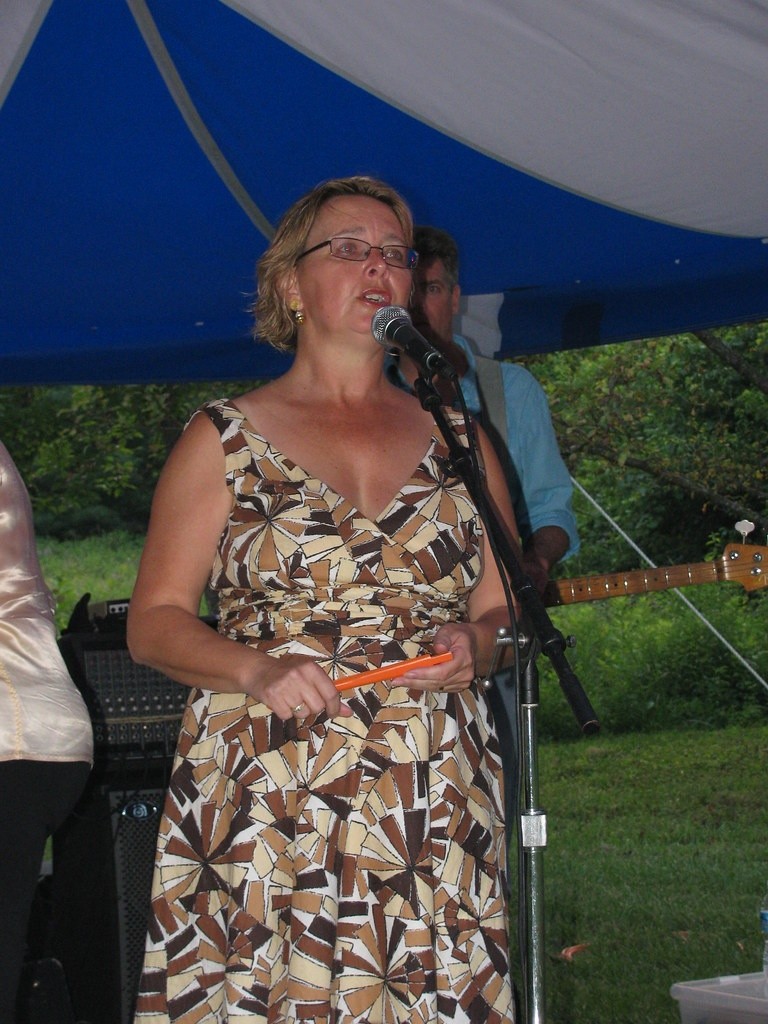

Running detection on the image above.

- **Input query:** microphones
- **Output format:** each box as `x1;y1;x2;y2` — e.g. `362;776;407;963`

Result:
370;305;459;381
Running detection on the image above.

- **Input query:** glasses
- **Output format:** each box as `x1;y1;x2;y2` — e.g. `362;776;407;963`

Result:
293;236;420;270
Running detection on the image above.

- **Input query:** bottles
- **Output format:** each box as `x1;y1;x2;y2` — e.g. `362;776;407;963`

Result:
759;888;768;979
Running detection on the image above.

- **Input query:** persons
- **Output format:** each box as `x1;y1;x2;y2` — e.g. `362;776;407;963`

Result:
0;440;96;1024
384;230;581;841
126;178;522;1024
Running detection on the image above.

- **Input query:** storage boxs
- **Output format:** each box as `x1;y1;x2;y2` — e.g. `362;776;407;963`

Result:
670;971;768;1024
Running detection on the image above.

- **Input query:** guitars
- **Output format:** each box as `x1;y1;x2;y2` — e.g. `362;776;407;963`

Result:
548;515;768;611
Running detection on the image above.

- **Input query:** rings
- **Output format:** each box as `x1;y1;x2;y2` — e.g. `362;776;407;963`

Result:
439;686;444;692
292;702;306;712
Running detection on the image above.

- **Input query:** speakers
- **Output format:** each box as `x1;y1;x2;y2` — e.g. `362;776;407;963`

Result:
59;776;169;1024
